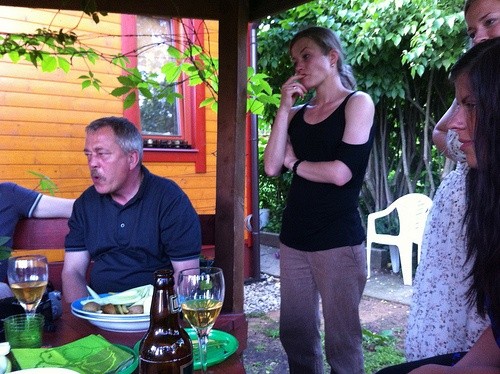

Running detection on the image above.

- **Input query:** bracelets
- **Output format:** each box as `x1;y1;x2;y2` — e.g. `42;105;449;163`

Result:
286;159;298;169
293;159;304;176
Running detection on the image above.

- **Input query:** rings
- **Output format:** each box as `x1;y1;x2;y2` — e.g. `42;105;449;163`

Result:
293;85;296;88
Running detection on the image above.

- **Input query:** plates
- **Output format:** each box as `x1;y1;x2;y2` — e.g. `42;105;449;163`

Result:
111;342;139;374
133;327;240;371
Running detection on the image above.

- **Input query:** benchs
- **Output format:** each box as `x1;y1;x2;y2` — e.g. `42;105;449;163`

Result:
10;214;217;293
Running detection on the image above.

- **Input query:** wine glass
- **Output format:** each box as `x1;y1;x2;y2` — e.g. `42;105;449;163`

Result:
7;254;48;317
176;267;225;374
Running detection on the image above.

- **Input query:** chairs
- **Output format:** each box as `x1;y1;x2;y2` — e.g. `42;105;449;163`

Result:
367;194;434;287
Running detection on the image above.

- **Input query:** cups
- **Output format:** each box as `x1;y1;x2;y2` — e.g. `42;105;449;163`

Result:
4;313;46;348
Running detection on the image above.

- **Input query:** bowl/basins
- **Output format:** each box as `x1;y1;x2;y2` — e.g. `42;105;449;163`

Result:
71;292;150;319
70;309;150;333
5;367;80;374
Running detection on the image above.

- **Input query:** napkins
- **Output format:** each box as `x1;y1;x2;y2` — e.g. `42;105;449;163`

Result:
9;332;134;374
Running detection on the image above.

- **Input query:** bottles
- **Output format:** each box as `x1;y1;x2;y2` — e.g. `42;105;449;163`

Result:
139;268;194;374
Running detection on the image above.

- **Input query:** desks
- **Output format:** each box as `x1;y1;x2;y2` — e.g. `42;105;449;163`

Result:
0;293;249;374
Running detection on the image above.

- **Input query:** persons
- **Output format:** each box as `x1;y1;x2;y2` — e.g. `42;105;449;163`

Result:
0;182;77;299
376;1;500;374
263;27;378;374
62;116;201;302
